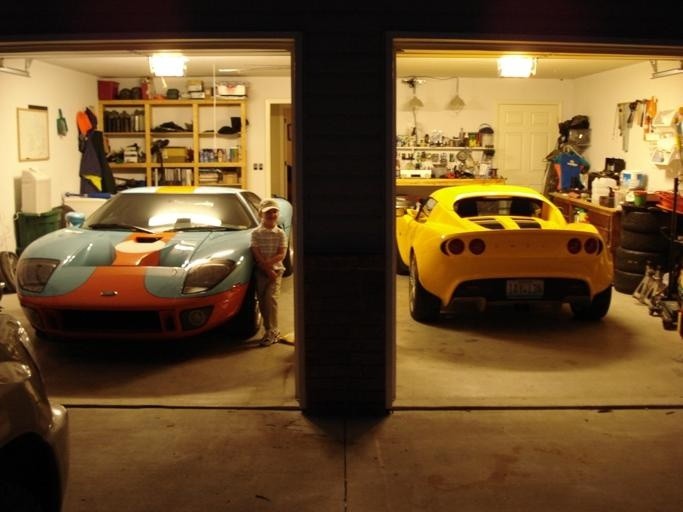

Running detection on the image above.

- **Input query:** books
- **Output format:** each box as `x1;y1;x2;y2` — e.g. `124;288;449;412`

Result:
152;167;224;187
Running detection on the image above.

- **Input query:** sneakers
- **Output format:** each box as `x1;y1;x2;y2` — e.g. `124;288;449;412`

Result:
260;327;281;347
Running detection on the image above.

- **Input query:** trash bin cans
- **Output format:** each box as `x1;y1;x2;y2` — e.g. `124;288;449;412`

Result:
15;208;62;256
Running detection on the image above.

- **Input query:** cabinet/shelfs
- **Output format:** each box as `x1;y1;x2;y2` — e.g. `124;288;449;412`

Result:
548;186;625;285
97;100;246;191
397;146;508;188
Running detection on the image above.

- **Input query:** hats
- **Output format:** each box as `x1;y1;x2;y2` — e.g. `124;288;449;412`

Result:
258;198;282;213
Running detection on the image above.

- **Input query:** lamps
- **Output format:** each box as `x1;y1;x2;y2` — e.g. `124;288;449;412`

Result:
408;77;467;109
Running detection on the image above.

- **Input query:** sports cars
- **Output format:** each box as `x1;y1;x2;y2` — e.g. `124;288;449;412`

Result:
392;185;615;325
14;184;294;357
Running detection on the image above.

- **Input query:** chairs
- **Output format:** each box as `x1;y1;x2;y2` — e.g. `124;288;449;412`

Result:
458;197;538;217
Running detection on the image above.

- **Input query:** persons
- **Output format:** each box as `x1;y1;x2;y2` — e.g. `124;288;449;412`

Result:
248;197;290;347
257;195;294;278
